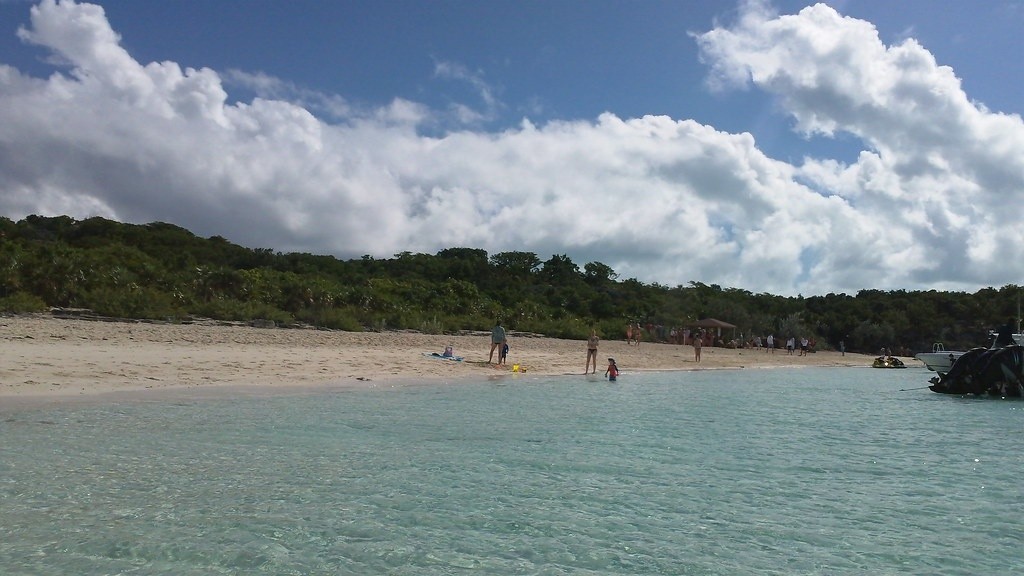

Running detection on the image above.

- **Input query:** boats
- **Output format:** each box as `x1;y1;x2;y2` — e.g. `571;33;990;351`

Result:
870;356;908;368
914;293;1024;374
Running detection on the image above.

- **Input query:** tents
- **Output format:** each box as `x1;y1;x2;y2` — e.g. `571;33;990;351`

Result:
686;318;737;338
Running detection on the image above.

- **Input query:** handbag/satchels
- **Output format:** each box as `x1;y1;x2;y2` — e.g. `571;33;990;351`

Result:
444;346;453;357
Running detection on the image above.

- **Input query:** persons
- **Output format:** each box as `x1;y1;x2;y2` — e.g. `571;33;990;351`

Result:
880;346;910;358
583;329;599;375
949;354;956;367
694;335;702;361
787;337;817;356
840;341;845;356
605;357;619;381
626;323;644;346
486;319;509;365
647;323;775;353
